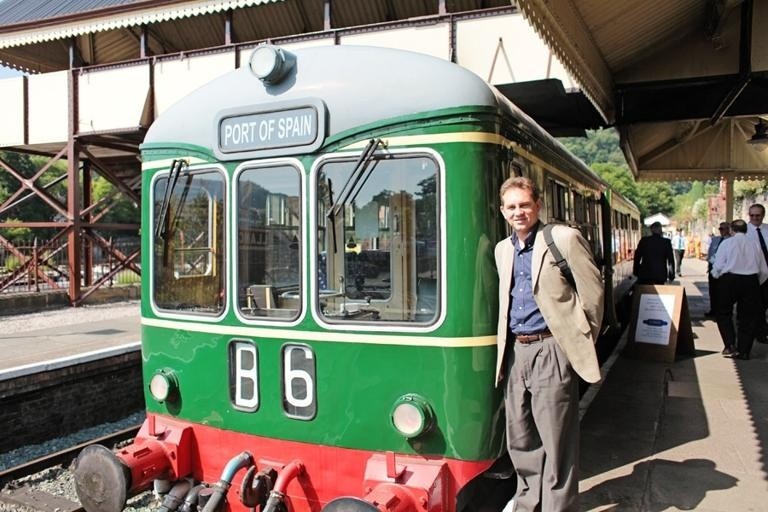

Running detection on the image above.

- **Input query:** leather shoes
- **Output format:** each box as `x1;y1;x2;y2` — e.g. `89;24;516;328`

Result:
722;344;752;360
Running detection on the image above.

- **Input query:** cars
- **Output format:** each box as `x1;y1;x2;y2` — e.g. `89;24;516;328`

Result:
51;262;114;281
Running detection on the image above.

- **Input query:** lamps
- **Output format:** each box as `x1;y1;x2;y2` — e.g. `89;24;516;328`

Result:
744;119;768;155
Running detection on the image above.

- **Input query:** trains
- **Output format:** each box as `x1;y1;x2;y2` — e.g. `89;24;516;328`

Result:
74;39;640;510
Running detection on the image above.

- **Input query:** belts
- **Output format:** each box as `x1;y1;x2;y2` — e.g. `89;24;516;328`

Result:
513;329;553;345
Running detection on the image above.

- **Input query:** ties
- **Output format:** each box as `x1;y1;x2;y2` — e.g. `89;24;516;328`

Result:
678;237;682;252
756;227;768;266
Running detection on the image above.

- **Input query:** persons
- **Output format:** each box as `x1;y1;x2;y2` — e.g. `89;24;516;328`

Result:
633;221;675;285
671;227;687;277
494;177;605;512
706;204;768;360
472;233;500;461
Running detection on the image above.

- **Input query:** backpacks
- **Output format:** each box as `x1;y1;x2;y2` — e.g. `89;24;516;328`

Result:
541;218;604;296
